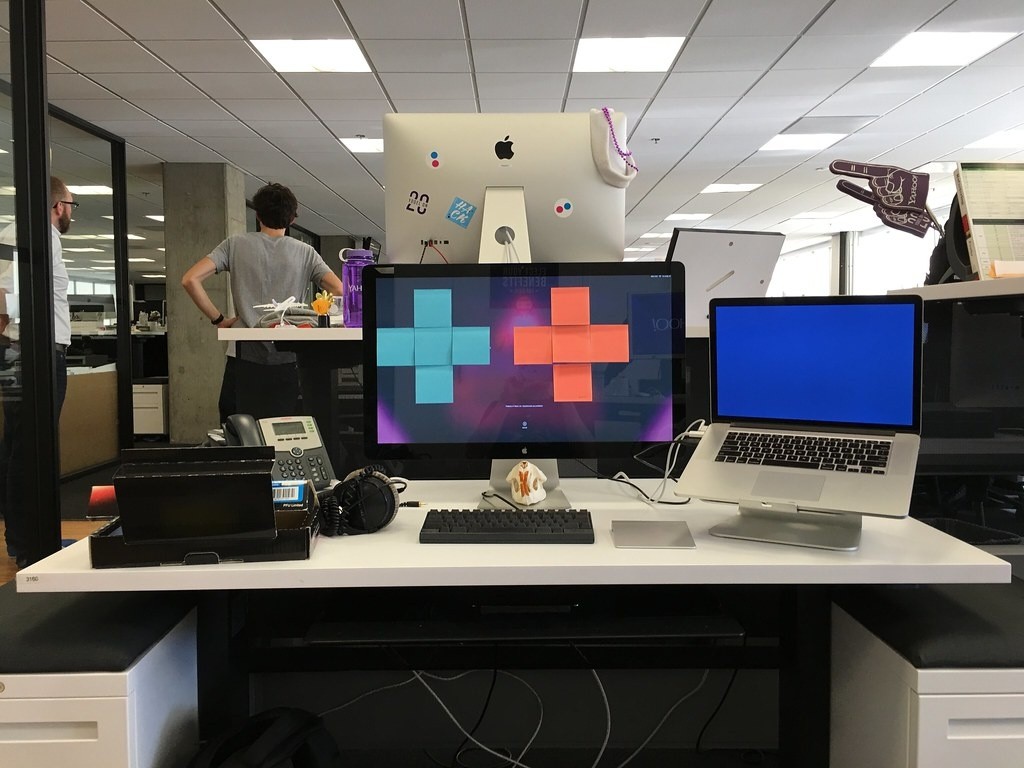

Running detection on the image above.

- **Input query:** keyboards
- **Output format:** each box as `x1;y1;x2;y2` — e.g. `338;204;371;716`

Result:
420;509;594;544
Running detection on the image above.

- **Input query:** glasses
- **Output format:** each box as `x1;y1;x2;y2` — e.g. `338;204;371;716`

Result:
53;201;79;212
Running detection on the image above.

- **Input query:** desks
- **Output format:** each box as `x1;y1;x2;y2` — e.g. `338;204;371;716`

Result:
71;324;167;374
13;477;1011;768
215;326;365;473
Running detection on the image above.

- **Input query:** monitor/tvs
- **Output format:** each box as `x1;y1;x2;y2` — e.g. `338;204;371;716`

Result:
886;278;1024;478
362;262;687;504
384;112;627;265
68;304;104;313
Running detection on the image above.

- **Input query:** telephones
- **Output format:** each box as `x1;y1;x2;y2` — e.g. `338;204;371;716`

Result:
222;414;334;490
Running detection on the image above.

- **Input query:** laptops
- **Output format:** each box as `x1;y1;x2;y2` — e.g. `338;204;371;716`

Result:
673;294;925;519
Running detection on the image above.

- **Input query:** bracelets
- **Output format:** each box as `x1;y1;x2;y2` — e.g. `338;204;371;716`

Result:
211;313;224;325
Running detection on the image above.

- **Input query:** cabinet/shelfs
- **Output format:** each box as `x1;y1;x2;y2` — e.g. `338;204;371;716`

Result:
0;578;200;768
132;377;169;440
828;575;1023;768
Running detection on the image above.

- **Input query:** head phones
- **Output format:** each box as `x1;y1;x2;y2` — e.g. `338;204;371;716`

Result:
313;467;400;534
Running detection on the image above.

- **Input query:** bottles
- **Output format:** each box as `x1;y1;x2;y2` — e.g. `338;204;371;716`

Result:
339;248;377;328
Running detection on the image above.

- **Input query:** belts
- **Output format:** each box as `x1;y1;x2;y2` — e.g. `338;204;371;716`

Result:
55;343;68;355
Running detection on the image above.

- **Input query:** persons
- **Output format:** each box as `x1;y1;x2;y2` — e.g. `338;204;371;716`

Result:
0;177;79;571
181;183;345;429
